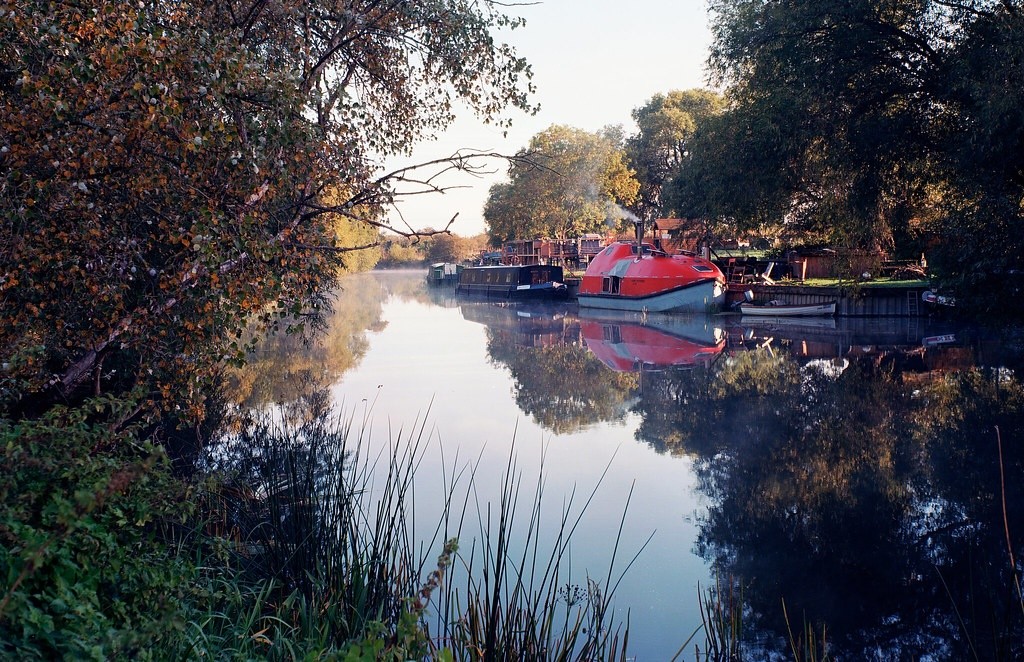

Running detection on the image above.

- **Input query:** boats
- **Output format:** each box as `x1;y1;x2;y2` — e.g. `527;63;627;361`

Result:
580;308;725;374
456;261;568;302
741;315;836;333
426;262;462;284
740;290;836;317
461;295;566;333
921;291;955;312
576;221;729;314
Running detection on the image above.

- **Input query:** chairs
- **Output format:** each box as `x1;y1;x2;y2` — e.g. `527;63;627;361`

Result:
731;264;746;284
